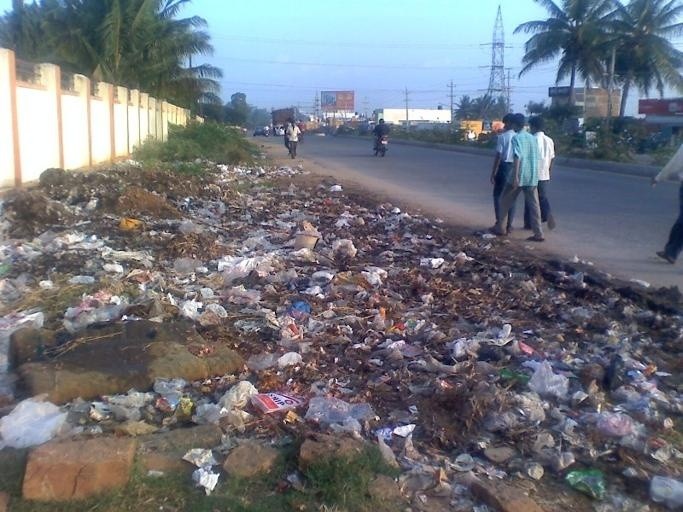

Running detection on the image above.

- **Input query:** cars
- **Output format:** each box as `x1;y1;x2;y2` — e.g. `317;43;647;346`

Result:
255;126;264;135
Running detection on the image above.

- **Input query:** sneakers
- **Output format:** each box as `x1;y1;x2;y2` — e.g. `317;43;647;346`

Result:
656;251;676;264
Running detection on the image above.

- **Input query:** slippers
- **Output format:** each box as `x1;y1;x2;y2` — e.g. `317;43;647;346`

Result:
488;210;556;242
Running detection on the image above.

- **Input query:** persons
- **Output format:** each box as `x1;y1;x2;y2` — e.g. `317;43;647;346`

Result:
285;119;301;159
522;116;559;231
489;113;520;233
282;117;292;155
371;118;391;152
651;146;682;263
487;111;545;242
297;119;304;143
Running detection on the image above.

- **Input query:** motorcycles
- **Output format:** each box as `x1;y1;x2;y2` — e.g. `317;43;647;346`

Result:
374;133;388;156
264;130;269;136
